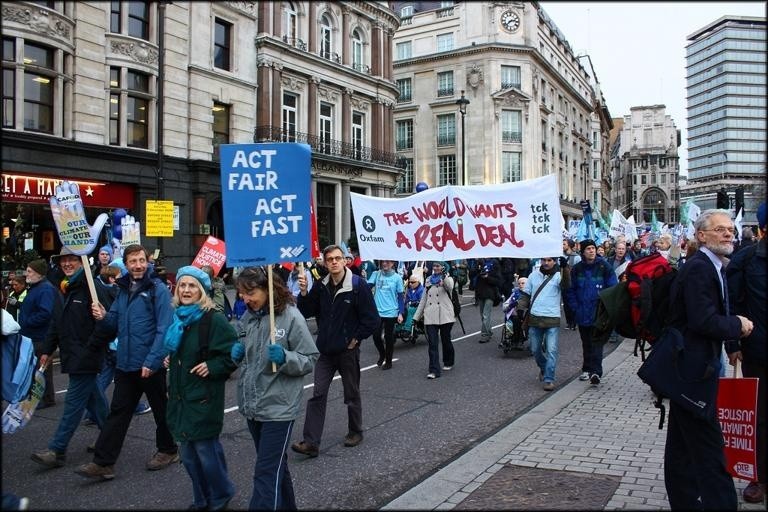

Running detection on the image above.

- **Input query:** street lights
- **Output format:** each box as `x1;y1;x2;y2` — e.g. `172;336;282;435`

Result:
455;89;471;186
580;156;589;201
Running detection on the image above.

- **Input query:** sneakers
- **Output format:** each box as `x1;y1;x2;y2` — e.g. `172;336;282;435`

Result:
31;400;179;479
292;440;319;457
378;326;618;391
344;432;363;446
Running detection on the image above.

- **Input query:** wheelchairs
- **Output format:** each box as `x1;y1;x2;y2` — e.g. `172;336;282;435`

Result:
392;315;428;344
499;295;545;355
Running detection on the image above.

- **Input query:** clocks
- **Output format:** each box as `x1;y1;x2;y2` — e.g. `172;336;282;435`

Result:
501;9;521;31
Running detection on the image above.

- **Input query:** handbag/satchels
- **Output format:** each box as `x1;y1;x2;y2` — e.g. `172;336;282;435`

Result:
716;376;760;483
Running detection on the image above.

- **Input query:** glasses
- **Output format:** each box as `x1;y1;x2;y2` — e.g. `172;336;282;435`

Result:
325;256;344;263
703;228;737;237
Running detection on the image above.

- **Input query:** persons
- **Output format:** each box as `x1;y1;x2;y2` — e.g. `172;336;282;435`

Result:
1;203;768;511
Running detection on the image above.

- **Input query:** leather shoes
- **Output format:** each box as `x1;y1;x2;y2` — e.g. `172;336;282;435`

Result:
743;481;764;503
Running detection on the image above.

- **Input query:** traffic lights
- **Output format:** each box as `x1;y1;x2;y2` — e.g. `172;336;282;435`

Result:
735;188;745;217
716;191;729;208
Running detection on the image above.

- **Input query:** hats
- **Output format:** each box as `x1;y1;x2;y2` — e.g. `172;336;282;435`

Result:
28;243;212;296
579;239;596;255
344;253;354;262
757;204;767;226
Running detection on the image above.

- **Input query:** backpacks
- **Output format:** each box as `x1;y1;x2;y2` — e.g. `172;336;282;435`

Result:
0;333;38;404
615;252;687;347
443;276;461;318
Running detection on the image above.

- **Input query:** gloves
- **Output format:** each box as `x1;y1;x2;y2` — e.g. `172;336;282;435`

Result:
231;342;245;363
441;268;446;277
268;345;285;365
